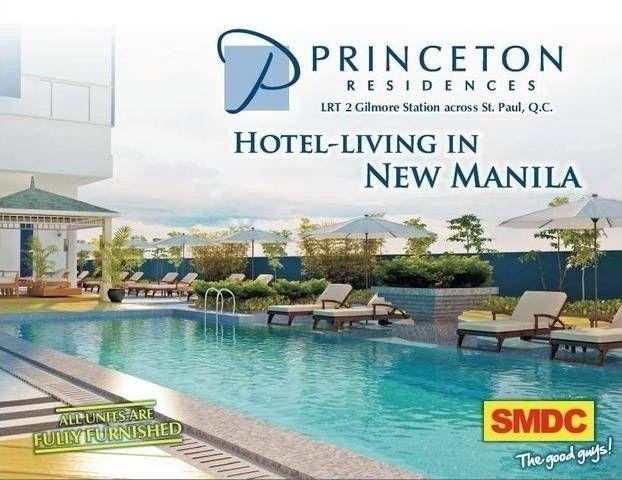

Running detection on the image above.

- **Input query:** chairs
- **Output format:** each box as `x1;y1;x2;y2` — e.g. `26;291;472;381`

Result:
76;269;144;293
456;290;571;353
143;272;199;298
186;273;246;301
312;295;409;331
267;283;352;326
255;274;273;285
127;272;179;297
549;303;622;366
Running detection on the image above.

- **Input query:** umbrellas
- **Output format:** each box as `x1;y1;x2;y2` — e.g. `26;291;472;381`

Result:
120;238;157;279
298;214;431;310
496;193;622;328
77;243;99;272
153;232;219;277
210;226;294;280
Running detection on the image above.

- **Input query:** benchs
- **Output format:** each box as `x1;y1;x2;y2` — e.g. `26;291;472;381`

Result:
0;271;19;296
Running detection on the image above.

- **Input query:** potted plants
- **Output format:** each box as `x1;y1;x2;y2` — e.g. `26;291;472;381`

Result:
94;225;142;302
23;235;59;288
372;251;499;322
